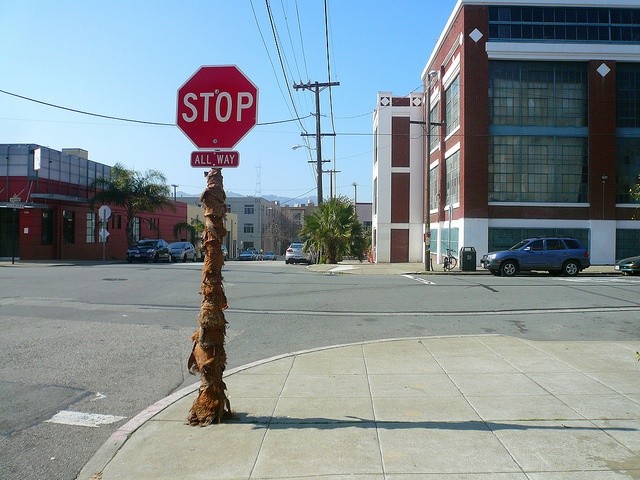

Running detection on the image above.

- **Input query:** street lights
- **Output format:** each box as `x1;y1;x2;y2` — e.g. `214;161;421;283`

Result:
292;143;323;204
352;184;357;216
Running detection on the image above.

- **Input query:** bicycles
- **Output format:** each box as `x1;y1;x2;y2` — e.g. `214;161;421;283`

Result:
443;248;457;272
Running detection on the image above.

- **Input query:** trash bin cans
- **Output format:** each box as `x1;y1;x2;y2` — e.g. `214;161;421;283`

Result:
459;247;476;271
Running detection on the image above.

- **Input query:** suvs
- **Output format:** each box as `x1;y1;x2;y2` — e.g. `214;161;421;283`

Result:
481;237;590;276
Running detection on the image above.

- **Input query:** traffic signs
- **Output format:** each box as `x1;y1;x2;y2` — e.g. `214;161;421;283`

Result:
191;151;240;168
176;65;259;149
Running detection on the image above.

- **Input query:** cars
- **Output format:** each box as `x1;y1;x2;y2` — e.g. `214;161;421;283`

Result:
170;242;197;263
252;250;262;261
615;256;640;275
127;239;171;264
286;243;312;264
263;251;277;261
239;251;256;261
